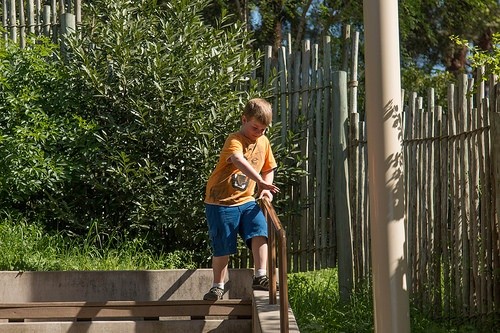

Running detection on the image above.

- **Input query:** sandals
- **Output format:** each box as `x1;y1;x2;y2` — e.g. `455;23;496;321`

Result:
205;286;224;300
252;275;280;291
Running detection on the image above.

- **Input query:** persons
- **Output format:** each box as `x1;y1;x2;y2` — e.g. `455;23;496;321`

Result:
202;98;280;301
